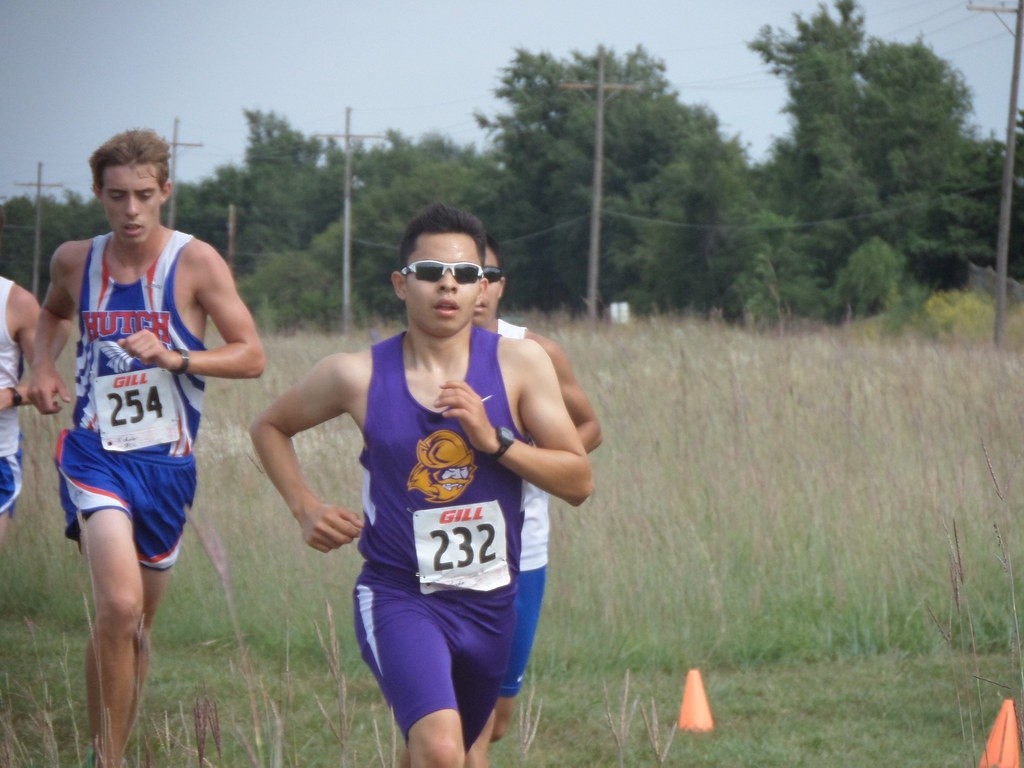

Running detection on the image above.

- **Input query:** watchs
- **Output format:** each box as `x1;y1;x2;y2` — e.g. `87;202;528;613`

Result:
491;426;514;461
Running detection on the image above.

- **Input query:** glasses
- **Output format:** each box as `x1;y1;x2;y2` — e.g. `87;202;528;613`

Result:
483;265;504;283
400;260;485;284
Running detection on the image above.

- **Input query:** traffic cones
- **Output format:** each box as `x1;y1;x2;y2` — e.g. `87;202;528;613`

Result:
977;698;1023;768
675;669;715;733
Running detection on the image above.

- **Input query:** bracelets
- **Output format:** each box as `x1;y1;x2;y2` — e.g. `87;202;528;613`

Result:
8;386;21;406
170;347;189;375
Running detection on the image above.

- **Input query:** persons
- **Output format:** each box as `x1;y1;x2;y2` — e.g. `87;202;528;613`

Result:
0;272;71;545
474;238;602;742
248;200;596;767
28;129;263;768
